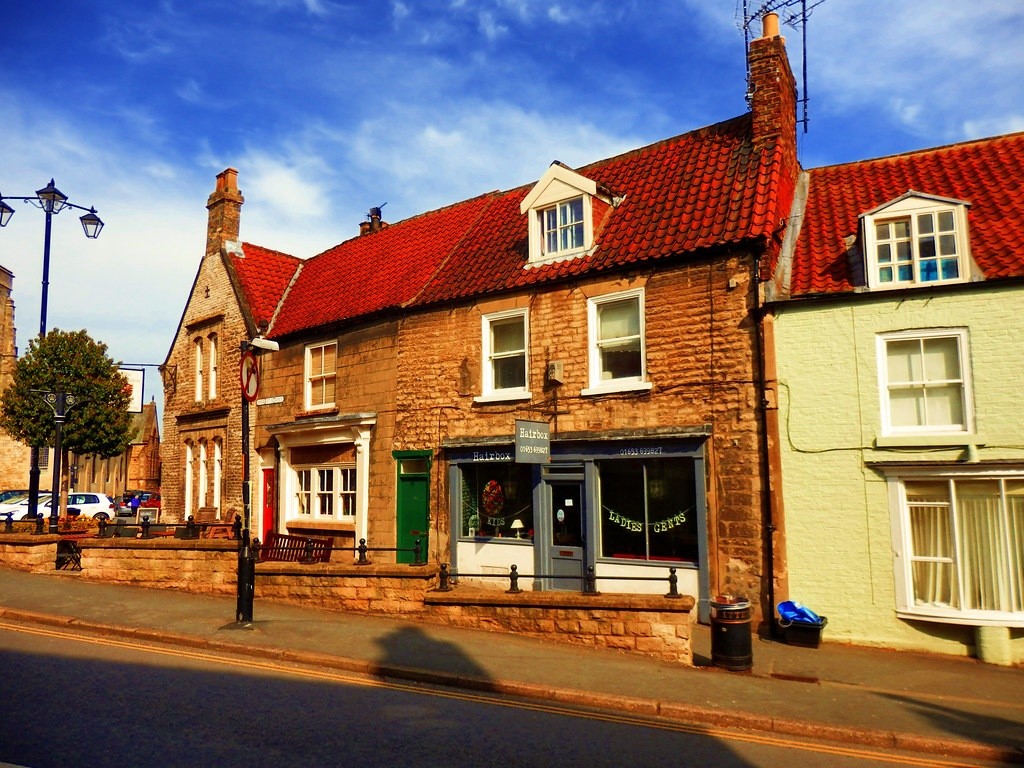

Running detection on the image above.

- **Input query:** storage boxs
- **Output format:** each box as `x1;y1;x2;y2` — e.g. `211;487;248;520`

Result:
783;616;828;648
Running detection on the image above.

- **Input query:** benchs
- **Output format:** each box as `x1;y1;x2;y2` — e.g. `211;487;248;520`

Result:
252;530;334;564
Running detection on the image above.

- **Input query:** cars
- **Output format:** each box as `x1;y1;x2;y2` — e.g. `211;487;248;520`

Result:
0;489;162;517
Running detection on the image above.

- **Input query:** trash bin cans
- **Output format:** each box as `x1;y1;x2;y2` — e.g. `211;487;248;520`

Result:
709;594;754;674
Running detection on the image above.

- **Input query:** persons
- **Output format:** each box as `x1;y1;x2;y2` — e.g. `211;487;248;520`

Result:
131;495;140;517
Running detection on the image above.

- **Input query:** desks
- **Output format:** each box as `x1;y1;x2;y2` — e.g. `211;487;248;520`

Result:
58;539;82;570
150;531;174;538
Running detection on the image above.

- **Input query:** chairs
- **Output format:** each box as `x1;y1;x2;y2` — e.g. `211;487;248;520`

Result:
105;507;237;539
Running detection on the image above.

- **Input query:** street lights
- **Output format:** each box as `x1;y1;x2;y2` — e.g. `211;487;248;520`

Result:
0;177;105;519
234;336;281;622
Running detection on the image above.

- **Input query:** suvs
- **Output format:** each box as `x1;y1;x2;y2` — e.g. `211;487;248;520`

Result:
0;492;117;522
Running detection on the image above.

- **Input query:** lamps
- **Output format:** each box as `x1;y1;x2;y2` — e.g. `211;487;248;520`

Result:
510;519;524;539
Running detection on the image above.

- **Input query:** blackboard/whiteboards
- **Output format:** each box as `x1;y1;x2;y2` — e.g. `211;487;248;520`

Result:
136;507;159;524
